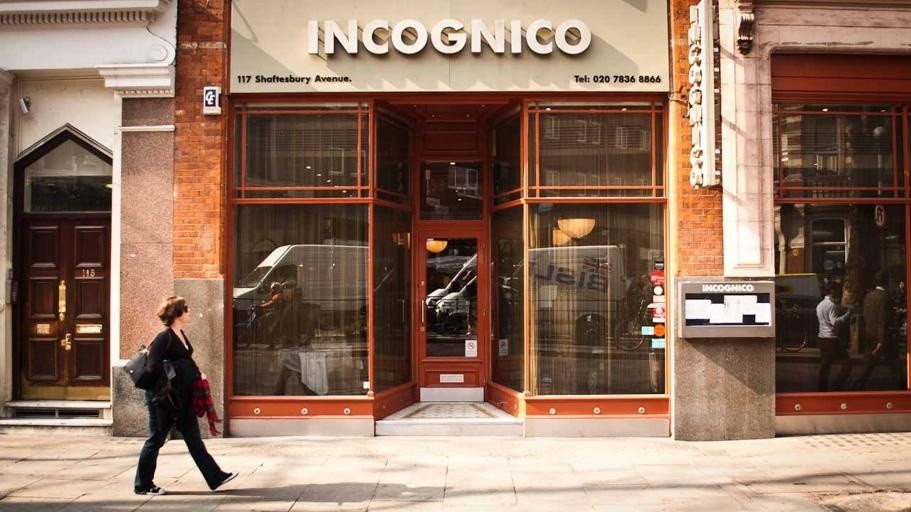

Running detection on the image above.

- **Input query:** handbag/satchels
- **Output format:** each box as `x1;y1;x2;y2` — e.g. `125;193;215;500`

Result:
123;345;169;392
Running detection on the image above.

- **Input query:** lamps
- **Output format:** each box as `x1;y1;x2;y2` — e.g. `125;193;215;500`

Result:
425;239;449;254
552;218;596;246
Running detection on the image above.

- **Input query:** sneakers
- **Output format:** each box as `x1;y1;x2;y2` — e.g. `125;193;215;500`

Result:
215;469;239;491
136;483;168;496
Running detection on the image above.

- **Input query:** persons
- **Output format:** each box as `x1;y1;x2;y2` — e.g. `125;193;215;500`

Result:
841;274;863;355
843;270;901;393
892;280;906;333
134;296;240;496
254;280;321;395
815;282;853;393
